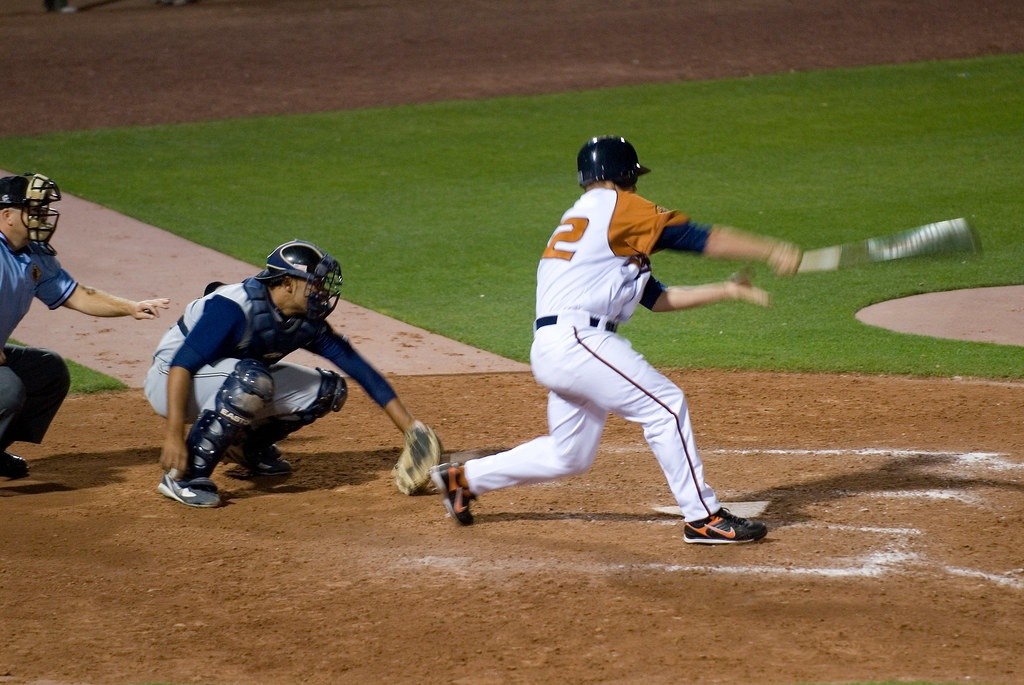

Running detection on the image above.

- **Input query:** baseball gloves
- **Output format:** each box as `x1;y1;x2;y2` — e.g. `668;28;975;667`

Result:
391;417;447;498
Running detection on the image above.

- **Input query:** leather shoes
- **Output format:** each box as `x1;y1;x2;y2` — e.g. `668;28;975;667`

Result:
0;451;30;476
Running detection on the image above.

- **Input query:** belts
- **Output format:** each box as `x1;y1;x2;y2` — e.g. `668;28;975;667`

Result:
534;315;618;333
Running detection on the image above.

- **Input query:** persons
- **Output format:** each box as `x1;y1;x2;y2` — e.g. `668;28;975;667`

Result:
0;173;169;479
144;240;441;508
428;133;803;545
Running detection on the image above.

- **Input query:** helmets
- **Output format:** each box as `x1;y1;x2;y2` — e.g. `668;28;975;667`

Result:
577;136;652;190
254;240;326;282
0;174;50;207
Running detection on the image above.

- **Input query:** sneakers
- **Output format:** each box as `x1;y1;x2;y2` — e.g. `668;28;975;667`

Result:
221;443;292;475
684;508;769;545
429;460;476;527
156;471;222;508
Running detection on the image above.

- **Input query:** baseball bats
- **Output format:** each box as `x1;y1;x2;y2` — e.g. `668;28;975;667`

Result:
794;217;984;274
